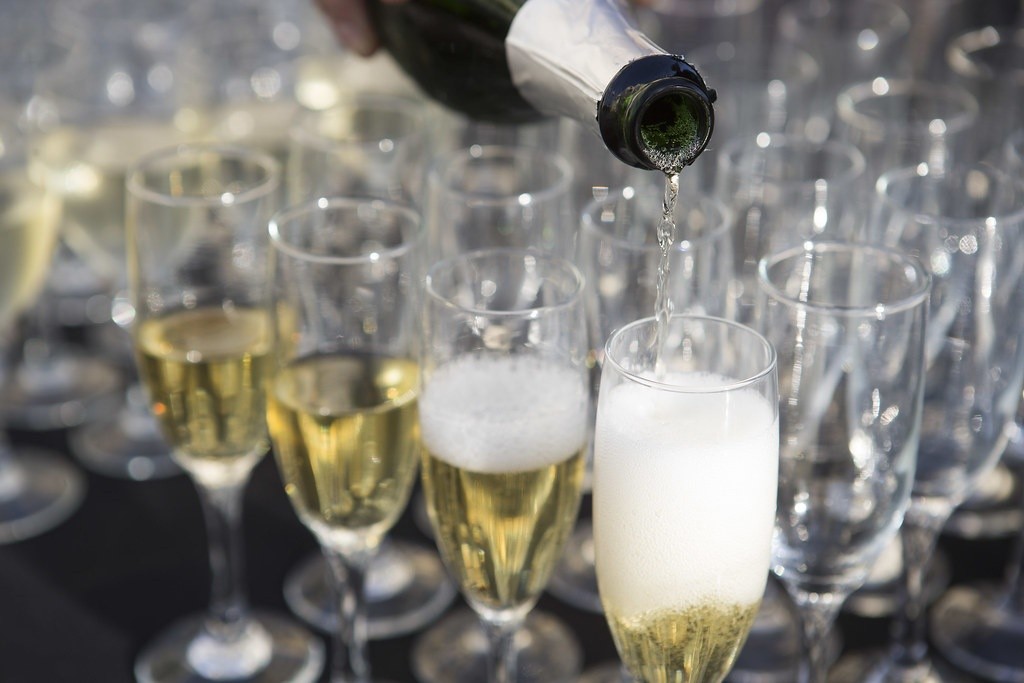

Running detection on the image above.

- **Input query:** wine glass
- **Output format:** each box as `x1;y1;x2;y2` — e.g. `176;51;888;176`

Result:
1;1;1022;682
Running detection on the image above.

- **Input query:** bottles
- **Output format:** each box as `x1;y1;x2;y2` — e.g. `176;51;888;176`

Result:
365;0;718;173
